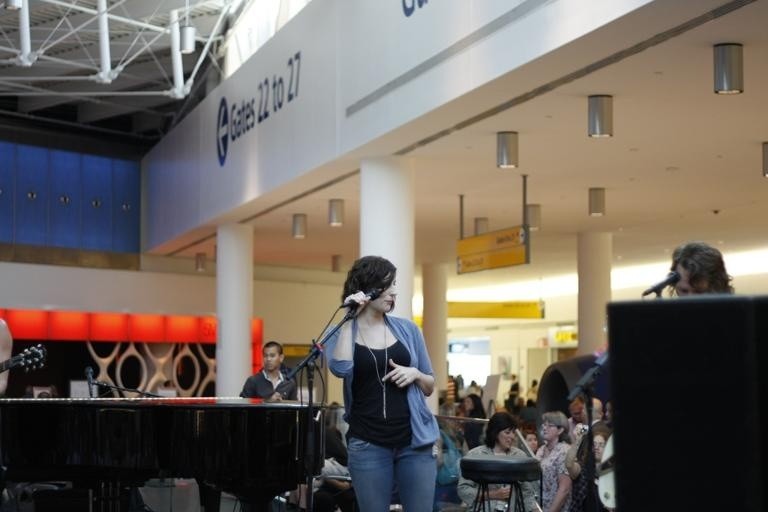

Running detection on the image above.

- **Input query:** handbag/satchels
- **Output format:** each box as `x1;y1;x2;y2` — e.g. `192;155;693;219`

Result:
437;429;459;486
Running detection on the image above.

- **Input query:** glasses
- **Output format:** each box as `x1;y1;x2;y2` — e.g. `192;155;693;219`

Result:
542;423;555;428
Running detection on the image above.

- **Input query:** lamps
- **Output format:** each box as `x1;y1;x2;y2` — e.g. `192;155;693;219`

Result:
497;131;519;169
589;188;606;217
762;141;768;177
713;42;744;94
332;254;344;272
526;204;541;230
588;95;614;138
194;252;206;273
475;217;488;241
329;199;344;227
293;214;307;239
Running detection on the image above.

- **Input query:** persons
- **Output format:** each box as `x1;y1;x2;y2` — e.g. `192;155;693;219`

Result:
239;340;298;402
668;238;736;296
325;255;441;510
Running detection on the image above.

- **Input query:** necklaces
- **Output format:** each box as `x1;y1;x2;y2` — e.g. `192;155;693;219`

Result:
356;319;388;420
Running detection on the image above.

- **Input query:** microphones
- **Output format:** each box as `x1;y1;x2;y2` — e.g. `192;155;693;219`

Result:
643;271;680;296
85;367;94;396
340;288;380;308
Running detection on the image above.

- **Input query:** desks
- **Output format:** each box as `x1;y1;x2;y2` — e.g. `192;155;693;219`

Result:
0;397;346;512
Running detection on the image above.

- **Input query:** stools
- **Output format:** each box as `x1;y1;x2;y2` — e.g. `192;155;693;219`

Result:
460;456;543;511
